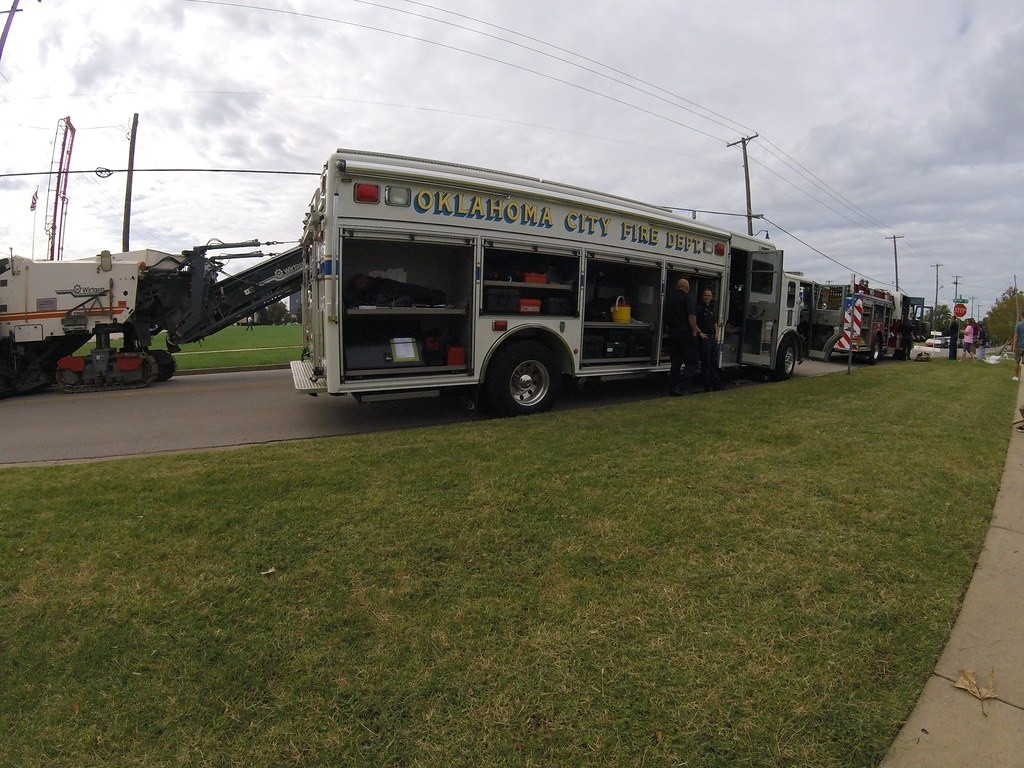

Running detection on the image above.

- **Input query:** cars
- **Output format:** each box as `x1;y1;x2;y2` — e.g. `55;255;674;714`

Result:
933;337;948;348
944;336;964;348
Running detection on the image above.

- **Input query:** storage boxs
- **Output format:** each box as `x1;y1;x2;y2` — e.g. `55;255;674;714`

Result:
519;297;541;313
524;273;548;285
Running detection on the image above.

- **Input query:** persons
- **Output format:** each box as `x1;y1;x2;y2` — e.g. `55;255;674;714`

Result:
971;318;980;358
899;320;914;361
958;319;976;363
1012;311;1024;381
696;288;728;391
246;316;253;331
977;324;985;358
665;279;697;394
949;316;958;360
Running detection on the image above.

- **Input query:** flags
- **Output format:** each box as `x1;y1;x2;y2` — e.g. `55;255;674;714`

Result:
30;190;38;211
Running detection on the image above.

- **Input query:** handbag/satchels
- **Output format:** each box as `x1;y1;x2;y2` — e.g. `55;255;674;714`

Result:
975;337;986;345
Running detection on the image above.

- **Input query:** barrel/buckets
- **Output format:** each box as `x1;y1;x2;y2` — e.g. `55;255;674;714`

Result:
611;295;630;323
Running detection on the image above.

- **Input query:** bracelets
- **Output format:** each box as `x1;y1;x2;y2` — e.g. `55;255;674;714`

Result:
698;331;701;334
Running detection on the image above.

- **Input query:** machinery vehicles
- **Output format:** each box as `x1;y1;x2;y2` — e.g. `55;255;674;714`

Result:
0;239;309;398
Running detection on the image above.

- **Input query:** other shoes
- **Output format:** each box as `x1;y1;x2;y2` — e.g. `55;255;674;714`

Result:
669;391;682;396
948;358;956;360
971;360;976;363
704;386;710;393
1011;376;1018;381
958;359;963;362
711;386;727;391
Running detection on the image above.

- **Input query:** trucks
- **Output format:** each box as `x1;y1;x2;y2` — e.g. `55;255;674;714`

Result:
810;280;934;366
290;148;822;418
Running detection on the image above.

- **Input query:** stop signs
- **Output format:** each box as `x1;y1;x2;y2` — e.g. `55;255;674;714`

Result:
954;304;966;317
